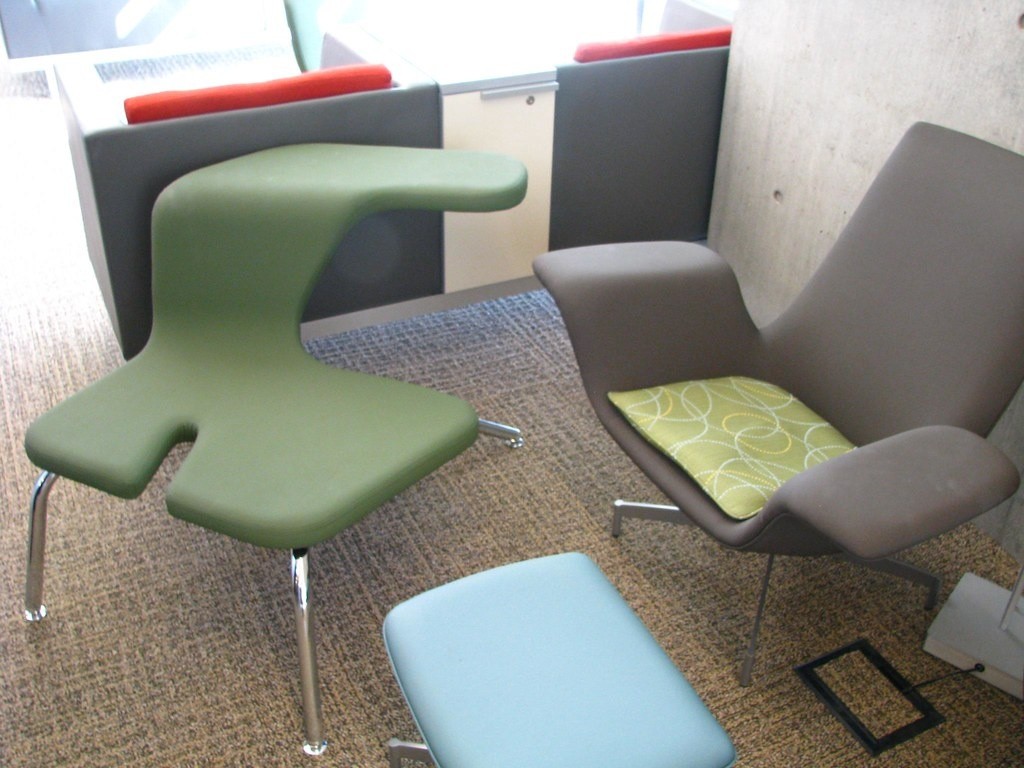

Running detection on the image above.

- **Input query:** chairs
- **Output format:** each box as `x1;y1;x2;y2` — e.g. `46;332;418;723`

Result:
19;142;529;759
531;122;1023;688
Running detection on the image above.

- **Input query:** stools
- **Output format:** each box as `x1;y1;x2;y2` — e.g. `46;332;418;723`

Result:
381;550;735;768
